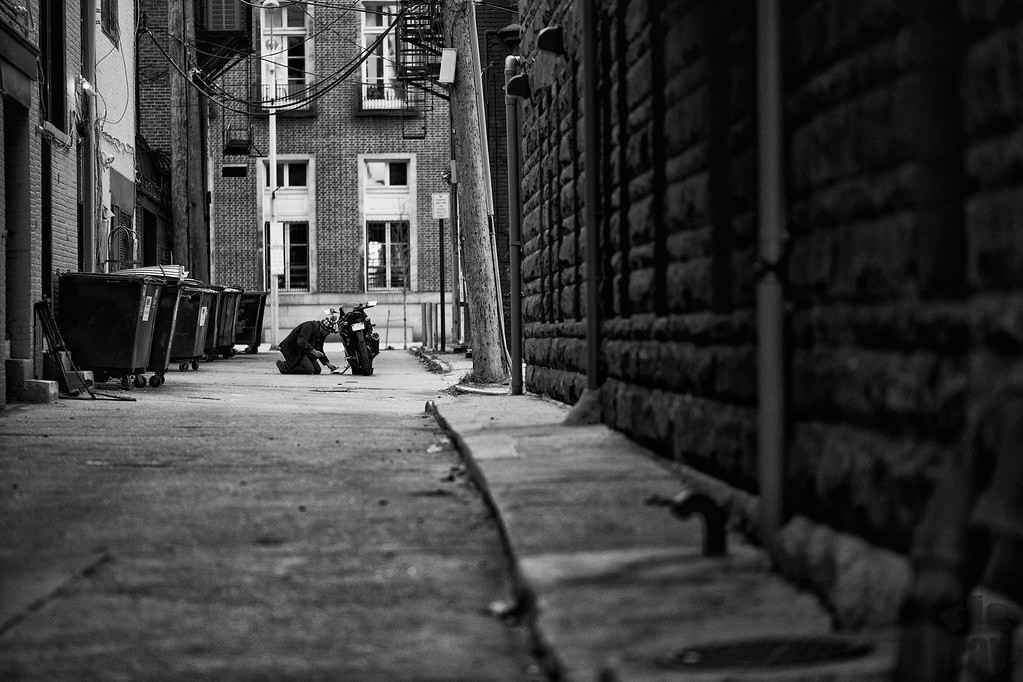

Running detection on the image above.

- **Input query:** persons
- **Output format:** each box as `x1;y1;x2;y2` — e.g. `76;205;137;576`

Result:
276;315;339;375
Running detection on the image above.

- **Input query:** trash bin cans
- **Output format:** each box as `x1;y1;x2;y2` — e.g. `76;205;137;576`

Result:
199;284;242;363
148;280;184;388
236;290;270;354
171;285;217;372
57;269;166;392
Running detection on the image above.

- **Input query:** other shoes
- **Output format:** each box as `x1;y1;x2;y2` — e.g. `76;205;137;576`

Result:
276;359;287;374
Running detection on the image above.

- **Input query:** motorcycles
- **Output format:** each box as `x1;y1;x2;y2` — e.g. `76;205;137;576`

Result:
323;300;380;377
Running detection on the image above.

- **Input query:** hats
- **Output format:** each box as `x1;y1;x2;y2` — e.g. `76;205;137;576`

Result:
320;315;337;333
